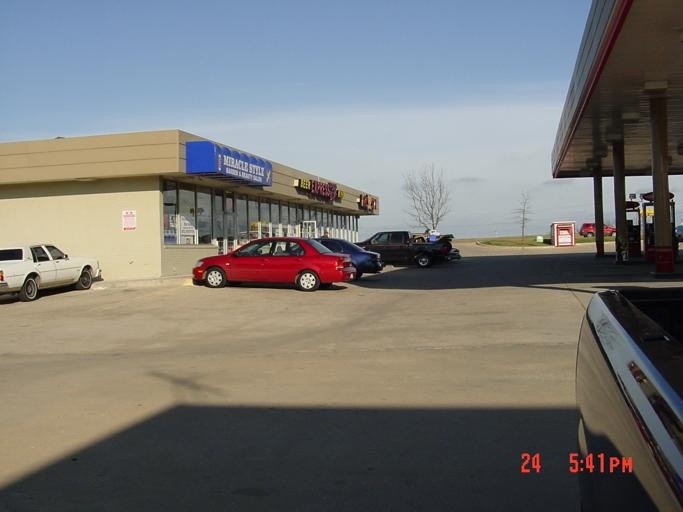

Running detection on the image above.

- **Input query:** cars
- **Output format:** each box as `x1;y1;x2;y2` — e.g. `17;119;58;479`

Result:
580;222;619;239
1;242;100;303
192;236;384;292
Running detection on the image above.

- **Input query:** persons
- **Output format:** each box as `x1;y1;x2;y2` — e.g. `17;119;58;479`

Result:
321;230;328;241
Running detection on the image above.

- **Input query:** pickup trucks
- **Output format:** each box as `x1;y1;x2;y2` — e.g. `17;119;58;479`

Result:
354;230;450;268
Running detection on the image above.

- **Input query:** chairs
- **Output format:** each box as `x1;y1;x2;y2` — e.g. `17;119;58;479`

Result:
275;246;290;256
